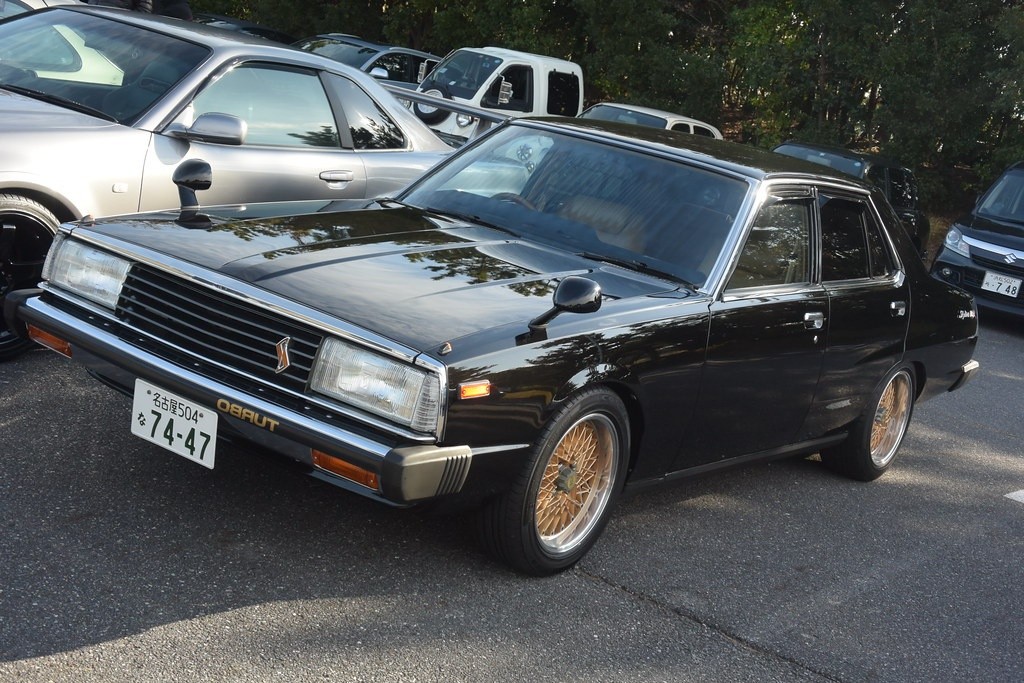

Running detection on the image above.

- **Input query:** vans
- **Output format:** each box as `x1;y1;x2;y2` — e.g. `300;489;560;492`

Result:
407;47;587;151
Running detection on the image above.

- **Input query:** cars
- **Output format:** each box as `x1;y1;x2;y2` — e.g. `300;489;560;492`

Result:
2;0;442;361
23;100;1022;579
